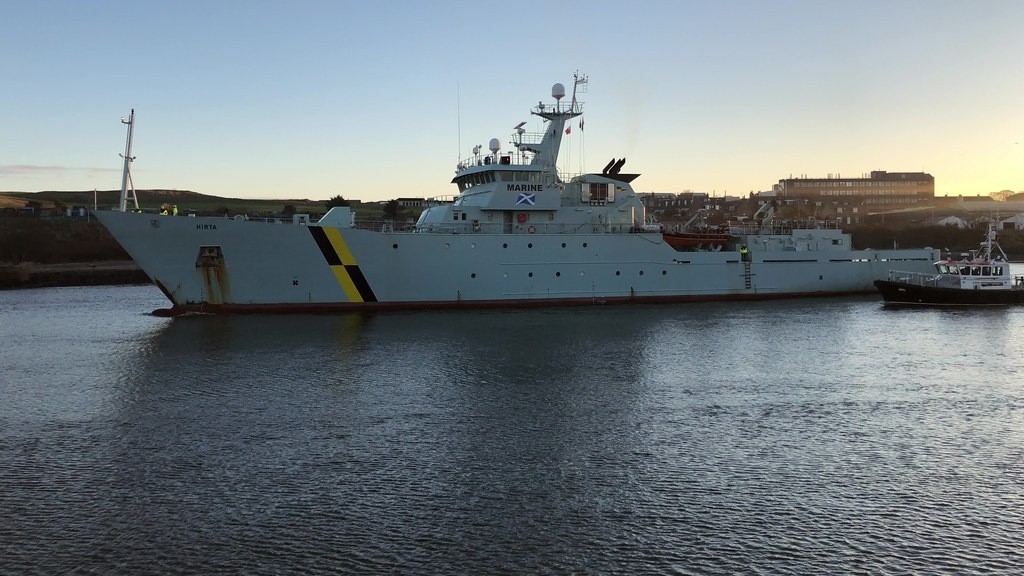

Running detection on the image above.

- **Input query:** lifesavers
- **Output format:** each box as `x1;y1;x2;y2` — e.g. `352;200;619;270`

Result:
528;225;537;234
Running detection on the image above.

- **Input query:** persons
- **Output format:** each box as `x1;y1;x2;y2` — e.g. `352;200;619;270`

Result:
1020;277;1024;285
740;243;748;260
547;180;563;189
485;156;491;164
756;219;802;236
126;202;178;216
997;253;1008;262
632;218;724;234
961;266;1002;275
410;219;481;233
697;241;715;251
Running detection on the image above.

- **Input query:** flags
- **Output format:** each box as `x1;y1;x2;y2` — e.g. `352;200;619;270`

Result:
579;123;581;128
581;120;584;130
565;126;571;135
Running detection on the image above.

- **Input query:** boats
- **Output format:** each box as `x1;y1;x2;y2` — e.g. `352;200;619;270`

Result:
872;201;1023;305
90;63;941;320
